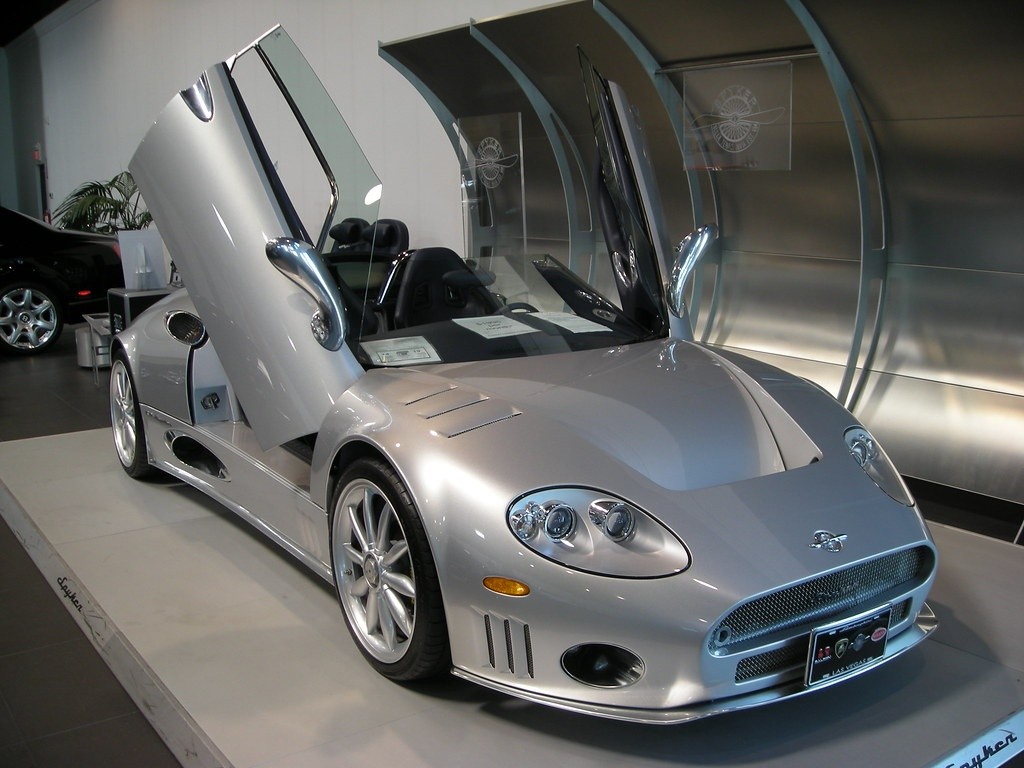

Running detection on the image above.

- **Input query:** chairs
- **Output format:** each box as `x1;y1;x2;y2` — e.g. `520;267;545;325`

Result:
395;247;502;329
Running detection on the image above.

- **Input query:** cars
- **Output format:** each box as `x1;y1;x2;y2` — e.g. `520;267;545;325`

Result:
0;205;124;356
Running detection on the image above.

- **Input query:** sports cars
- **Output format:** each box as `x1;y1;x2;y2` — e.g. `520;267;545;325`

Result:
100;22;939;730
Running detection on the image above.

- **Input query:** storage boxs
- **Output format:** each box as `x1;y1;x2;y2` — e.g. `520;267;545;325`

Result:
106;288;178;335
